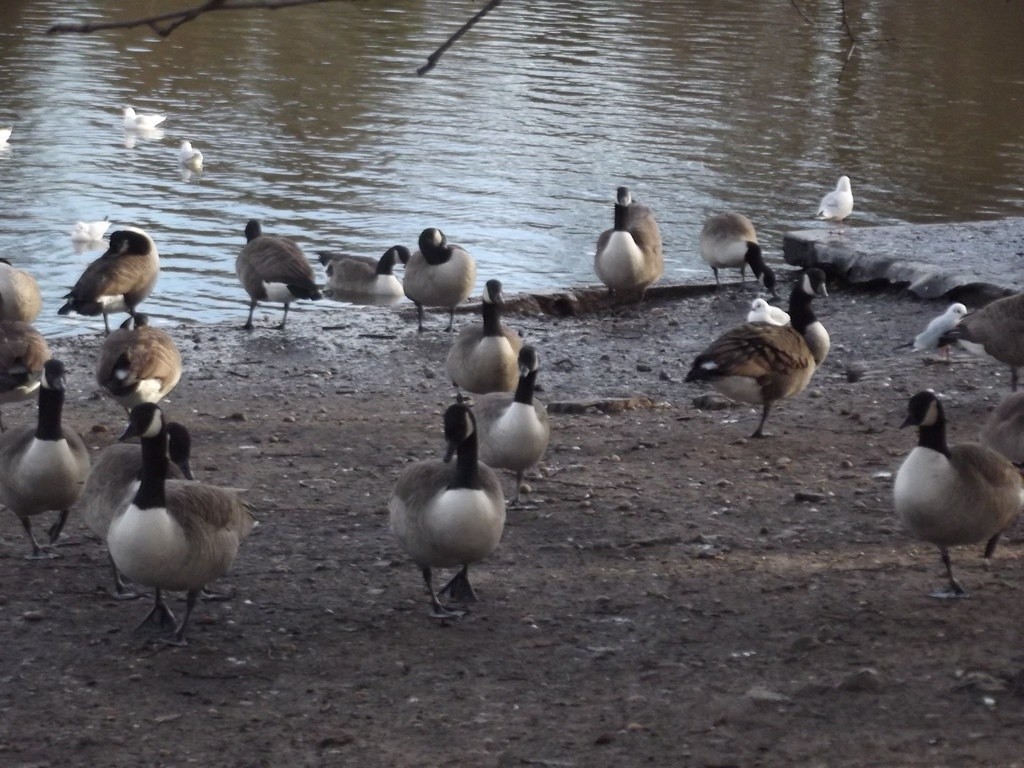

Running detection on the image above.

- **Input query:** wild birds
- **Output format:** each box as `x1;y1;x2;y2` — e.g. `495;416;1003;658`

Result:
71;221;112;241
593;182;664;319
0;219;550;647
894;291;1024;598
685;212;832;438
816;175;854;235
890;303;967;365
180;141;203;163
122;107;167;128
0;123;17;151
